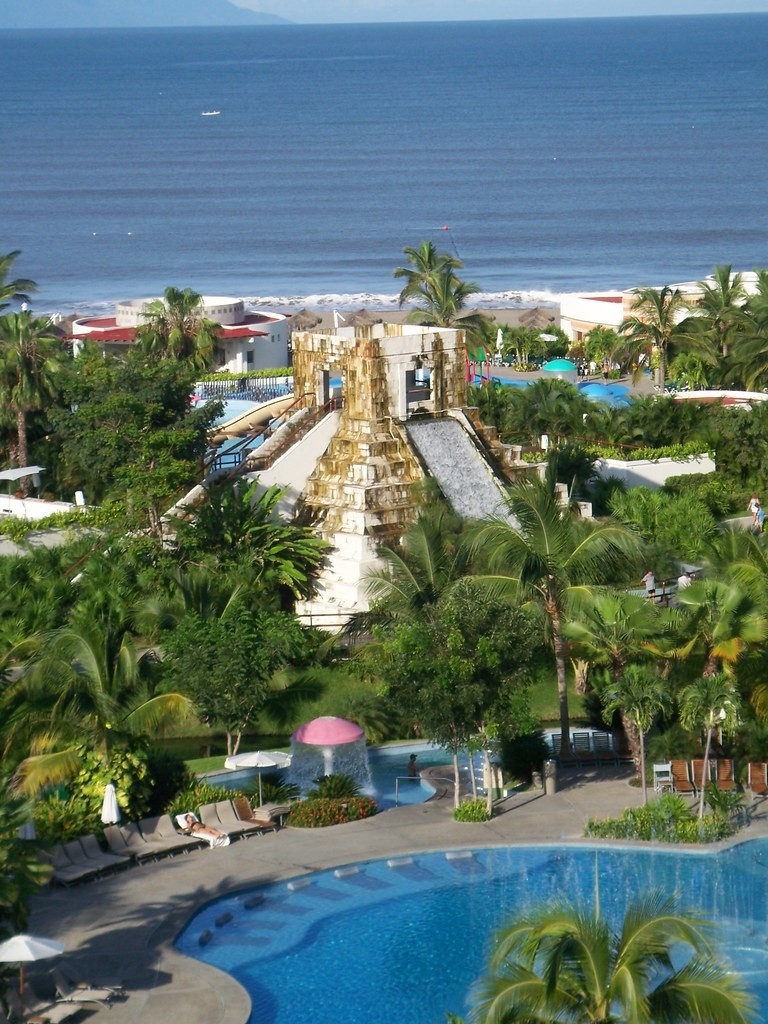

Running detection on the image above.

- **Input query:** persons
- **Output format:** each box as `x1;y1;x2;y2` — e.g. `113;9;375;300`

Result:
407;754;418;782
184;814;226;839
576;359;597;383
677;572;692;593
746;492;760;526
639;570;656;606
757;506;766;533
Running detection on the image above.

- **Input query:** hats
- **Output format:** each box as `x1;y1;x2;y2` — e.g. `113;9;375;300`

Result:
410;754;417;758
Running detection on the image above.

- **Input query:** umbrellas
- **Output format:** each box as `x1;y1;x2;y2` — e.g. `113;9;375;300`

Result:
533;333;559;342
224;750;293;808
496;328;505;354
0;934;65;1001
18;815;37;841
100;784;122;855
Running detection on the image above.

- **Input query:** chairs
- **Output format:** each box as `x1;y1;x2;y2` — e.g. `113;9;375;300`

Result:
0;959;125;1024
41;795;310;892
652;758;768;801
551;730;636;770
